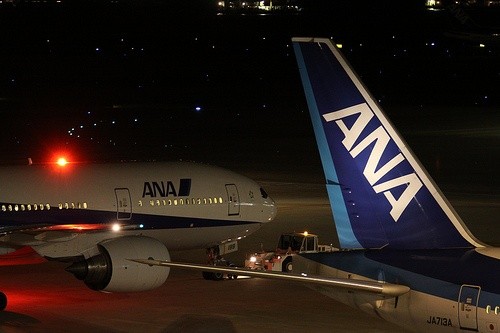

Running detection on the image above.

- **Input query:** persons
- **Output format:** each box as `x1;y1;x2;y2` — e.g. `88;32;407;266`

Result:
208;248;215;266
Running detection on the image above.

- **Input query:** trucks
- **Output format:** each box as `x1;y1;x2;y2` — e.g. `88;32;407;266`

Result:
244;230;340;274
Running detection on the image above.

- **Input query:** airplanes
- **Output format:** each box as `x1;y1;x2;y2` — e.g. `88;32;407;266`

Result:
1;162;409;314
125;36;499;333
